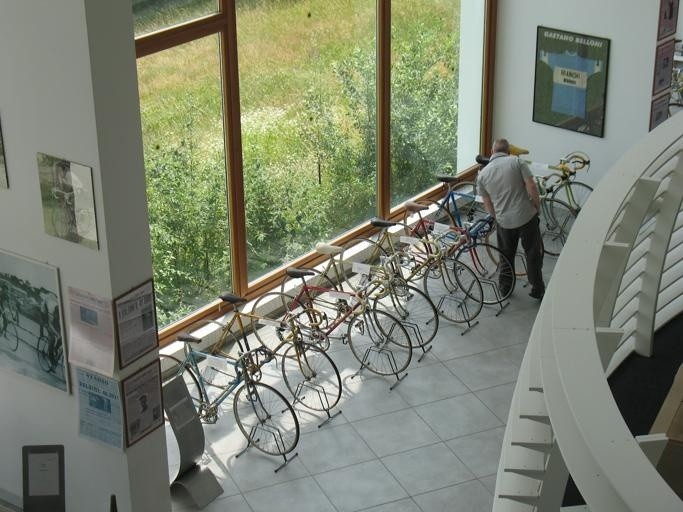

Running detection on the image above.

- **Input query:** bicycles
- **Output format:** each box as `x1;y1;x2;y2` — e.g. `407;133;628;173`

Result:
1;307;20;352
45;188;94;240
36;333;68;384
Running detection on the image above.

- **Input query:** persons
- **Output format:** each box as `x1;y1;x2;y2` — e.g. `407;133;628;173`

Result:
477;137;547;298
54;164;86;228
0;283;62;372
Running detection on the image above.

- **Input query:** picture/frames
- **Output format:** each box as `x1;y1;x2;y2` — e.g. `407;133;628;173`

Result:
653;39;675;96
0;250;71;397
658;0;679;42
649;92;671;132
532;24;611;140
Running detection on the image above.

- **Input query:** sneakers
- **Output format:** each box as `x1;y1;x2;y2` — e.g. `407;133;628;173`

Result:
528;287;545;300
